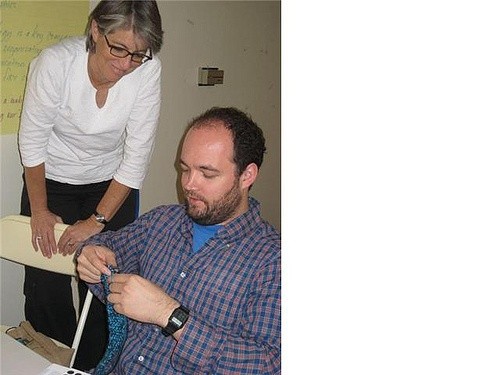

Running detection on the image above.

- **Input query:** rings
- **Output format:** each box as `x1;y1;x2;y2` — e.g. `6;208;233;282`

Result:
36;236;43;240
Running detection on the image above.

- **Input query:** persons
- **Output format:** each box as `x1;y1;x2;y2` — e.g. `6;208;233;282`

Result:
73;107;281;375
18;0;164;372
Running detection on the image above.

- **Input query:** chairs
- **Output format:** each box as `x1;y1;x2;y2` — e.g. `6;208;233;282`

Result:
0;214;93;368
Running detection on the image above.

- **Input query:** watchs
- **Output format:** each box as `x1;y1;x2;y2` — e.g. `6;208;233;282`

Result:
93;211;108;225
161;304;190;337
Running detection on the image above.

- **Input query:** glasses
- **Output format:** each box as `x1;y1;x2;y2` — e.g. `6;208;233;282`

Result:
104;34;152;63
